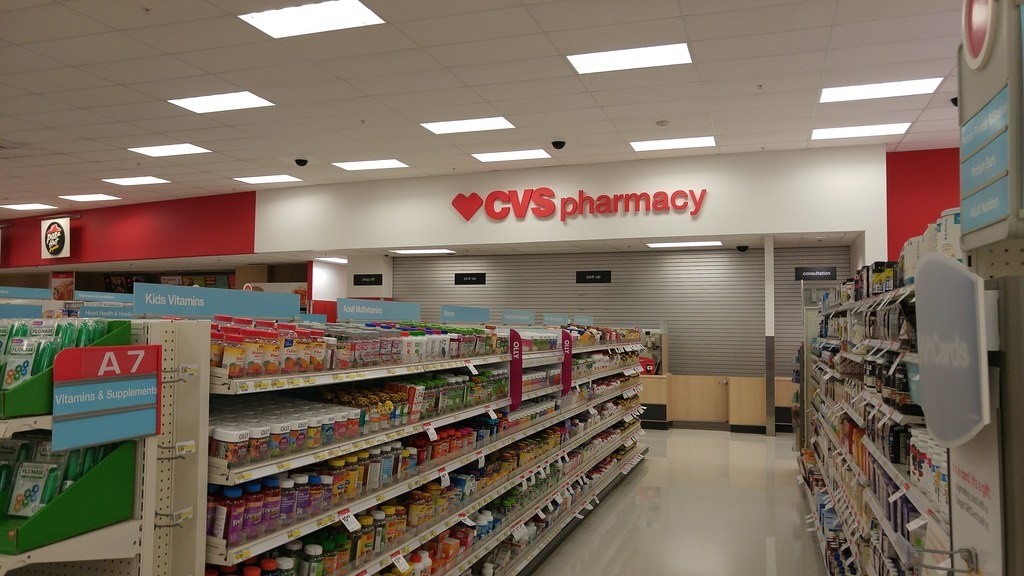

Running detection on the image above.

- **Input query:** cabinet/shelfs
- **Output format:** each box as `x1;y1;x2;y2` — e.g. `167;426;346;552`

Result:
804;285;1024;576
0;319;652;576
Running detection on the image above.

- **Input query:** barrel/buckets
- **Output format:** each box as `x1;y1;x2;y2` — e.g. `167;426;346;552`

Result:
904;206;962;285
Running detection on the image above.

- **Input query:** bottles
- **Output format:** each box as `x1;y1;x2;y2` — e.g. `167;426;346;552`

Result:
205;416;579;576
911;428;950;525
210;314;558;378
207;396;561;549
863;349;923;416
208;368;561;469
870;531;906;576
825;533;859;576
563;349;640;509
558;323;639;347
